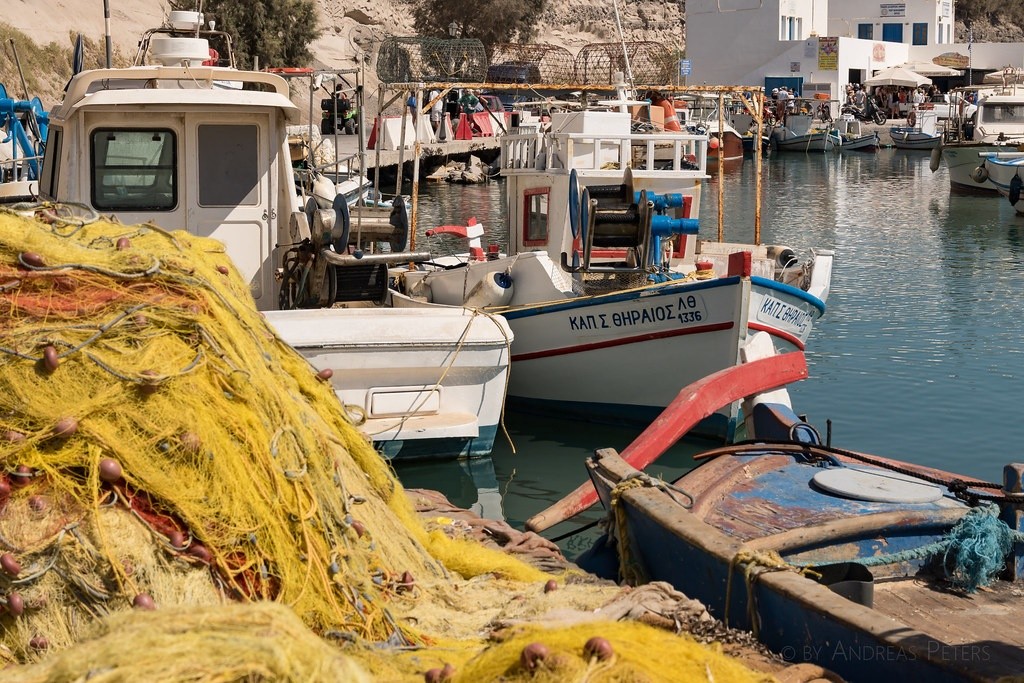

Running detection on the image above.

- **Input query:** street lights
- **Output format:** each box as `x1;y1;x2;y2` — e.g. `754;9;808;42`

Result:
959;22;973;86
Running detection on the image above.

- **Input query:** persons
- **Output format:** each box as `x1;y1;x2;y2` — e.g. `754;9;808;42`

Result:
406;88;481;133
756;86;800;126
846;82;941;125
331;84;347;99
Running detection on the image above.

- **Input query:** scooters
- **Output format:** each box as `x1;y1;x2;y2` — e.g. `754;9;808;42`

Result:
817;101;832;122
840;92;887;124
742;101;776;127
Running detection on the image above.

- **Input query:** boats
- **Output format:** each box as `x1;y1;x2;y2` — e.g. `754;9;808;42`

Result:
582;397;1024;683
0;0;1024;462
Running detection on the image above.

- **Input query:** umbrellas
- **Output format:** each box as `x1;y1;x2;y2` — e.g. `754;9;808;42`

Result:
863;66;932;93
